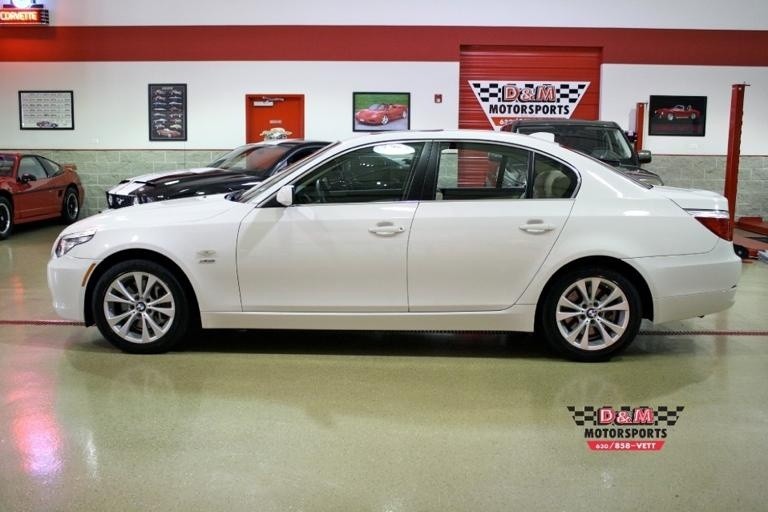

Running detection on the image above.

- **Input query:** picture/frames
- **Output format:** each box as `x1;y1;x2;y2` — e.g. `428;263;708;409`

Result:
148;84;187;141
353;93;410;132
19;91;74;129
649;96;707;136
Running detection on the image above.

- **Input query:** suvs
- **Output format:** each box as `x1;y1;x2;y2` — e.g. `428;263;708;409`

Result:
485;120;663;188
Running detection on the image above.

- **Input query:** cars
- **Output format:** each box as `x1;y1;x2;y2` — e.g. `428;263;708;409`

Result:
0;151;83;238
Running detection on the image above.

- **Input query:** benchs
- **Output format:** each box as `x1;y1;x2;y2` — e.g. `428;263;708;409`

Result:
519;170;570;199
